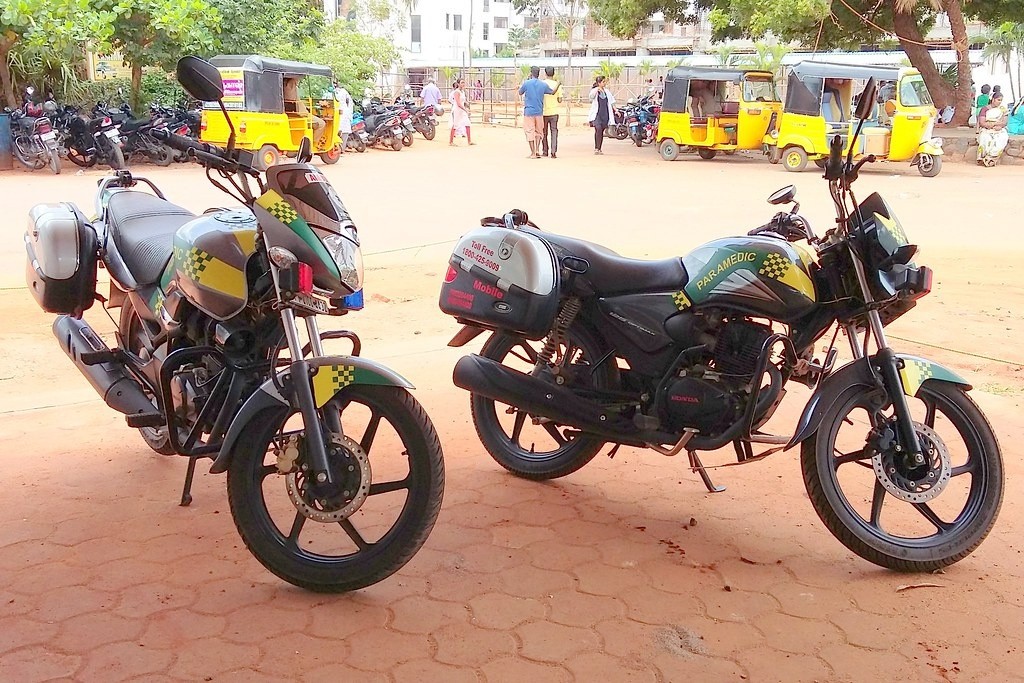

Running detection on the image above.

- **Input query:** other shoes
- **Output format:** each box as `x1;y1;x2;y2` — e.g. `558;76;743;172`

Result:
542;153;547;157
449;142;457;147
468;142;476;146
595;147;603;155
551;154;556;158
527;153;542;159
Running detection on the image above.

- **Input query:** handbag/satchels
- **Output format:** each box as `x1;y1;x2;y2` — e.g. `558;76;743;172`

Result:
941;106;956;125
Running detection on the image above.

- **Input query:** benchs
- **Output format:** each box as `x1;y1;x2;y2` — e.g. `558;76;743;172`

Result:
688;96;710;124
822;93;849;134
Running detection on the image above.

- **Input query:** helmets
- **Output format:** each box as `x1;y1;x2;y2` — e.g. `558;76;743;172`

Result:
435;103;444;116
44;101;57;115
27;101;42;118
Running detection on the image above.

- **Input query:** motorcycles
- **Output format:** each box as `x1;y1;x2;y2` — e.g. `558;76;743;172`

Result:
22;54;446;595
776;61;946;178
201;54;344;168
655;65;779;164
607;72;666;150
5;84;71;173
55;82;446;172
438;75;1006;574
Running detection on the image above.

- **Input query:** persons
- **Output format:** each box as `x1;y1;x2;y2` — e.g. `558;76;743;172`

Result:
448;79;477;147
542;67;563;158
876;80;894;104
394;92;415;105
473;80;482;100
588;75;621;155
418;78;442;116
282;76;327;151
1006;95;1024;135
970;85;1009;168
518;66;561;158
324;80;353;153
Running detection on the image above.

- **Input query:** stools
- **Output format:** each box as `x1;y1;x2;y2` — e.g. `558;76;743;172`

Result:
715;113;737;127
862;126;891;135
284;100;307;117
